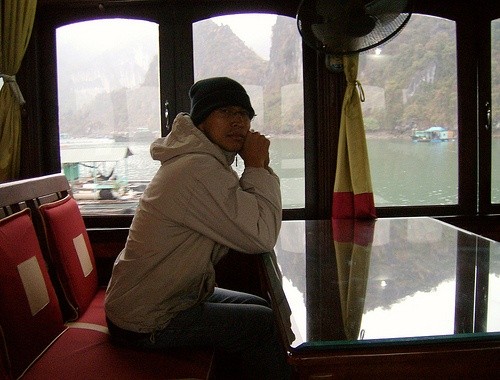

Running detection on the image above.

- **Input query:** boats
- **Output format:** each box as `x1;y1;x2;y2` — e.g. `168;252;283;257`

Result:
62;158;152;207
409;125;457;144
60;133;78;146
113;126;155;144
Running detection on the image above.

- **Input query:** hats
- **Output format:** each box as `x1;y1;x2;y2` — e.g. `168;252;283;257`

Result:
190;77;255;126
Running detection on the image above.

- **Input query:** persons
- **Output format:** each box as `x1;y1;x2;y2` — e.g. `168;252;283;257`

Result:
104;77;287;379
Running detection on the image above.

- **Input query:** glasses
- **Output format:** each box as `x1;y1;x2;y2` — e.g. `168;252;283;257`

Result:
214;110;256;122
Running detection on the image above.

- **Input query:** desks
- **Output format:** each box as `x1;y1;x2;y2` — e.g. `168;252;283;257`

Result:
254;215;500;380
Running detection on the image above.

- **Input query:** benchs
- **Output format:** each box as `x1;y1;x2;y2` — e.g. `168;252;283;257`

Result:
0;173;153;380
